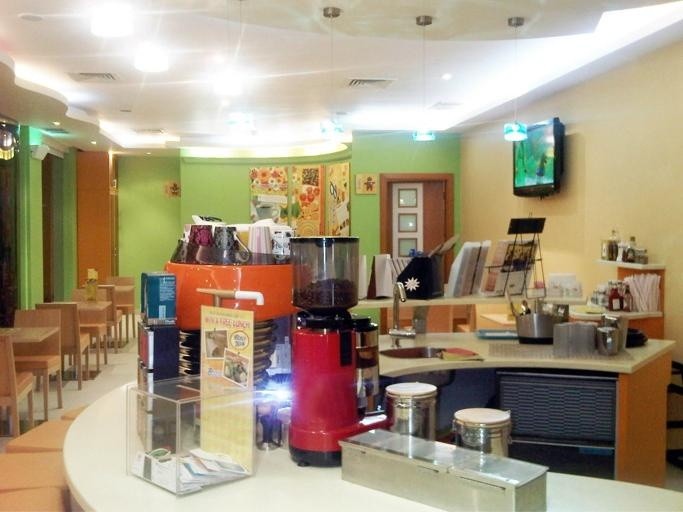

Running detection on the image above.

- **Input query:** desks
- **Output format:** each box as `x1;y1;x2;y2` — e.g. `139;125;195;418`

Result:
0;325;58;343
357;284;586;331
51;300;112;312
118;285;134;292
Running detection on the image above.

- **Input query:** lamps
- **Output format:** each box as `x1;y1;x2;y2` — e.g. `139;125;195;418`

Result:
504;17;528;143
412;15;436;143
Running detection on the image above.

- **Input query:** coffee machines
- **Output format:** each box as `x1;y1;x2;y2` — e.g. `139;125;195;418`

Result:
287;236;392;468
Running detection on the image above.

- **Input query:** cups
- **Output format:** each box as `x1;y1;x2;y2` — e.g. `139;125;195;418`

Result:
186;225;214;265
247;226;287;265
214;226;250;264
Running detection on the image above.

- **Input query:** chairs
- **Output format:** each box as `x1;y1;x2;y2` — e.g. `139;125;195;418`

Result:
82;283;122;348
109;273;137;343
36;303;90;390
12;309;63;420
0;334;35;437
72;289;108;364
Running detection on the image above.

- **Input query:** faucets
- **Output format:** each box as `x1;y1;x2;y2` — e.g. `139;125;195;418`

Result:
388;282;416;349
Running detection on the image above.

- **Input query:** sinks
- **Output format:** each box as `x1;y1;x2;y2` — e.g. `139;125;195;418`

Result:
379;345;447;360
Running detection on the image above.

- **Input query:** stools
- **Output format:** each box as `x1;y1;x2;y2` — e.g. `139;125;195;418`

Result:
7;421;74;451
1;453;69;493
0;488;66;512
62;404;89;420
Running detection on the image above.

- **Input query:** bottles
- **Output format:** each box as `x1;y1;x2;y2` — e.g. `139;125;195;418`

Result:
622;285;633;312
625;236;636;263
606;281;623;310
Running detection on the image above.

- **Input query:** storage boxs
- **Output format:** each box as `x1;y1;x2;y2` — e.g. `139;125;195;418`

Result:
127;372;256;495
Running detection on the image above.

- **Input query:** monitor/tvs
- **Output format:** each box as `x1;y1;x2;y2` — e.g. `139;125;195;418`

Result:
512;117;564;198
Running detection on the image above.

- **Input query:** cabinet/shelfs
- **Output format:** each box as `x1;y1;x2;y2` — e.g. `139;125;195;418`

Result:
593;254;665;339
